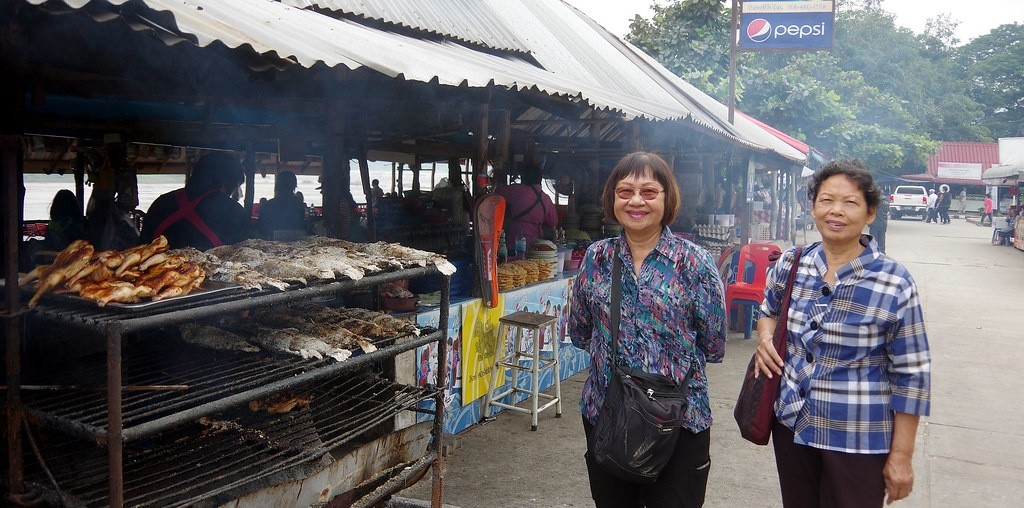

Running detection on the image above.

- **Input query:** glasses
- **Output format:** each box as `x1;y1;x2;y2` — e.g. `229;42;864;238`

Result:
614;186;665;200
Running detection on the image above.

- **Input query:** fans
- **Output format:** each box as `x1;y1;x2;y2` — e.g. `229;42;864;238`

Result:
543;144;589;198
487;126;534;171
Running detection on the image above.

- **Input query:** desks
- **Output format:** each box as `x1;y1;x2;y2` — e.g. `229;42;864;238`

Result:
378;267;591;436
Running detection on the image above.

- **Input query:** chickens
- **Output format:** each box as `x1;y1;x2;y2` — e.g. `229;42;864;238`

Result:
18;234;204;309
252;394;313;415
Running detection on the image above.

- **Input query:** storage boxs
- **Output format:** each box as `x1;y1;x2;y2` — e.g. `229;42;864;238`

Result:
375;187;468;252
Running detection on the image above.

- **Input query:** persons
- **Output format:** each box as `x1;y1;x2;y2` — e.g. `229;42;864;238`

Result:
371;179;398;209
868;189;889;252
568;151;727;508
258;171;309;239
924;186;951;224
994;209;1020;245
754;159;930;508
958;187;967;214
977;194;993;226
45;189;130;251
135;151;252;337
499;174;559;257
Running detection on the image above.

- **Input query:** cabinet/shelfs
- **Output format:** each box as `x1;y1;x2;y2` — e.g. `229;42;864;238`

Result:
0;262;451;508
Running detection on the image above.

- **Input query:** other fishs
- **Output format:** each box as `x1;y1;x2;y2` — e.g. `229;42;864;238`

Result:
220;301;418;363
178;322;260;352
171;231;458;293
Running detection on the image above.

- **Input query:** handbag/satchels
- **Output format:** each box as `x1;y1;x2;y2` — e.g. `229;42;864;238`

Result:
734;319;787;445
591;364;688;484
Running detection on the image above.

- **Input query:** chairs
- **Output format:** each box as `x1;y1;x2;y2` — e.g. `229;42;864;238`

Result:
725;243;781;339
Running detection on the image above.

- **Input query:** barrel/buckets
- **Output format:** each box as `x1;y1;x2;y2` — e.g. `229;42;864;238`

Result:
560;249;573;270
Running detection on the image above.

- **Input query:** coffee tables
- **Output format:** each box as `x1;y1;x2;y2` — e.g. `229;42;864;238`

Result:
481;310;562;432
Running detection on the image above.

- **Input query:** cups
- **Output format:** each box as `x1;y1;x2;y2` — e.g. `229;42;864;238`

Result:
557;253;565;273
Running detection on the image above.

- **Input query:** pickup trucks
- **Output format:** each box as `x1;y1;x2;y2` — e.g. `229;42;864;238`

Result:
889;186;928;219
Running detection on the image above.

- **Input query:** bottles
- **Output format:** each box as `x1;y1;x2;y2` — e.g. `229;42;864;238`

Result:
515;231;526;260
498;233;507;262
555;229;567;247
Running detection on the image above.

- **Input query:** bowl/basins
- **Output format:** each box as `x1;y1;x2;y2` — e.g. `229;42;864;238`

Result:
382;296;418;311
571;260;581;269
